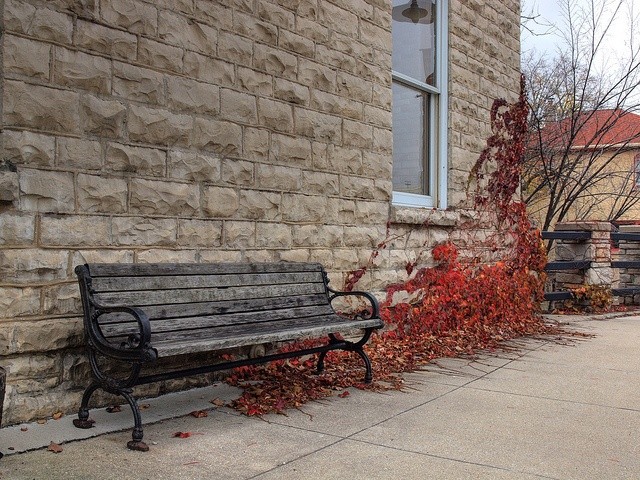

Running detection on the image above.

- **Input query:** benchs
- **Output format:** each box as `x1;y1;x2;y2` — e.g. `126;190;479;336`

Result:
75;263;385;450
